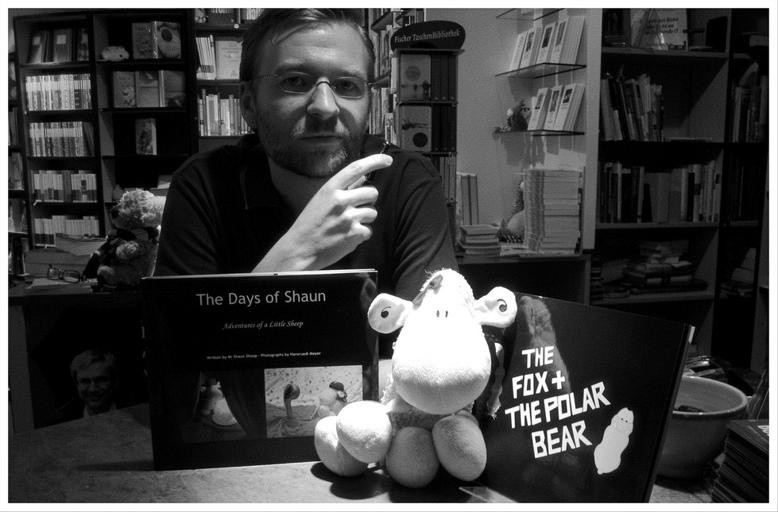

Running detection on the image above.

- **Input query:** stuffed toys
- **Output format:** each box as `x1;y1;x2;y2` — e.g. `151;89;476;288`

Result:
82;189;165;286
314;268;518;489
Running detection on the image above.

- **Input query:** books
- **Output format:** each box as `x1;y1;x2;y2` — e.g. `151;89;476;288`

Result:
363;10;769;291
9;11;260;258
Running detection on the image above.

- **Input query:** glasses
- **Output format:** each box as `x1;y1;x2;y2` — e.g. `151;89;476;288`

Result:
248;67;373;101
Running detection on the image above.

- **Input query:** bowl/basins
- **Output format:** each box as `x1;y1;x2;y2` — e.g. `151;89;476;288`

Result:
657;374;747;477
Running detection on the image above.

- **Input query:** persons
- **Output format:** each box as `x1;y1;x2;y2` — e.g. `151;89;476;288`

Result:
45;346;153;473
154;9;462;351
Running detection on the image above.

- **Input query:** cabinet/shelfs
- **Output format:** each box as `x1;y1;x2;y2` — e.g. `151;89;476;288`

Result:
487;9;769;367
8;9;465;280
